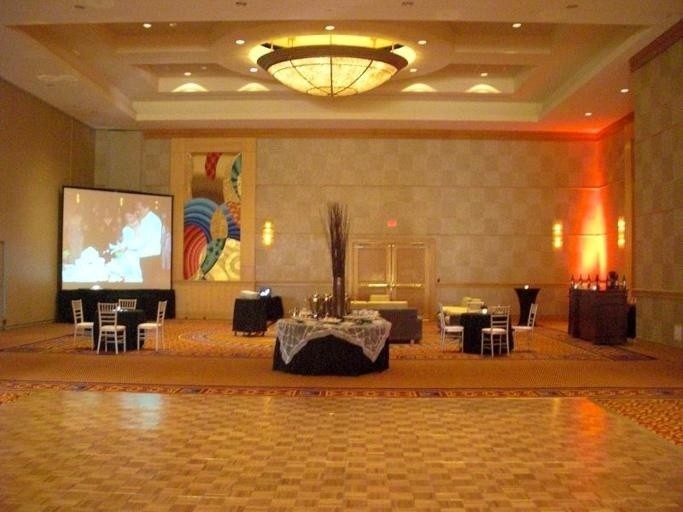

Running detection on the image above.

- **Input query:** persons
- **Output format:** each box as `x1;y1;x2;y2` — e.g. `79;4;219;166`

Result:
62;192;171;284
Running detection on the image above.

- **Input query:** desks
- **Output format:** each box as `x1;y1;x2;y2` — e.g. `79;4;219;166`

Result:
272;318;393;376
233;298;268;336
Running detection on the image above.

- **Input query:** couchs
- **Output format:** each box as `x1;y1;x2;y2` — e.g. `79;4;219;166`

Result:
436;296;488;339
370;294;390;302
347;301;423;345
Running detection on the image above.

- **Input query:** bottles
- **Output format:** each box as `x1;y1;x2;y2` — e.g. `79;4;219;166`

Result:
568;272;627;291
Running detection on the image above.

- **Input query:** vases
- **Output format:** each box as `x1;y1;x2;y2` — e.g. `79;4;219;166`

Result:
333;276;345;318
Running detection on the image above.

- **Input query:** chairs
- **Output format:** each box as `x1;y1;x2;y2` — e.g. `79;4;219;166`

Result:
439;303;539;360
71;299;167;355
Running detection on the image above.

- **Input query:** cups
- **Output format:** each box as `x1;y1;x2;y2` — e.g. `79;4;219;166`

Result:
287;293;379;325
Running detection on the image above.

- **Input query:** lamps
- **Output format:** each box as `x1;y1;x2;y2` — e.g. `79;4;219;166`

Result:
248;34;417;97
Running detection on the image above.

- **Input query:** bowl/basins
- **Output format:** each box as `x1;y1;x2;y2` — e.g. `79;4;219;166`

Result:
239;290;258;300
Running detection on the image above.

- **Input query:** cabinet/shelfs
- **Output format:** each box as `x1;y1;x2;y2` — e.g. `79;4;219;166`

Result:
568;288;628;345
